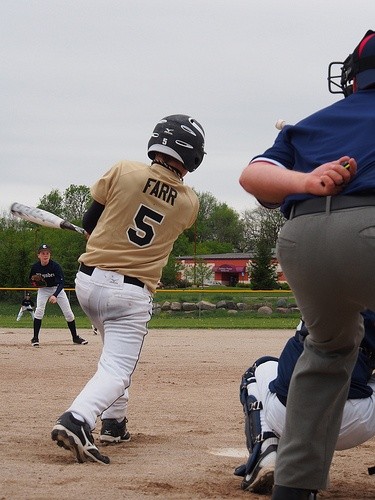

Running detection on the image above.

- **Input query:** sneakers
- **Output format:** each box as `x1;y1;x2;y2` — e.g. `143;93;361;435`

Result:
99;417;131;443
240;444;278;491
73;335;88;345
31;337;40;346
51;411;110;465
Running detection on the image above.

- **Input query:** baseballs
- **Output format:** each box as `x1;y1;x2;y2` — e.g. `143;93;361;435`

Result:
275;119;286;130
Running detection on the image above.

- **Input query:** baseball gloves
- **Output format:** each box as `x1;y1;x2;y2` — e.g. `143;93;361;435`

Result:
30;275;47;288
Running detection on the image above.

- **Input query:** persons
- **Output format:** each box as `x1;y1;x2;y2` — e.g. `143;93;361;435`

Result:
238;29;375;499
16;292;35;321
29;245;89;346
236;304;375;494
50;114;208;466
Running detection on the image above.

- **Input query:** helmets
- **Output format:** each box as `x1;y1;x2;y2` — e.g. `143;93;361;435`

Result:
147;114;207;173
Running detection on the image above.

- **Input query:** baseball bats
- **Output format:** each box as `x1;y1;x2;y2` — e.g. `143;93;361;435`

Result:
10;202;85;235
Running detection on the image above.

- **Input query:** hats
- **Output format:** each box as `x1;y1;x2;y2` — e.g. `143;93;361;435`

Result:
38;245;51;253
356;31;375;62
26;292;31;296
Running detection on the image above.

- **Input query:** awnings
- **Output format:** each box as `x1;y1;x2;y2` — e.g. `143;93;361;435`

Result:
212;266;245;273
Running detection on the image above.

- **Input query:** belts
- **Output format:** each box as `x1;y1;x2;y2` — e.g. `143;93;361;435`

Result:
288;195;375;218
79;261;144;288
